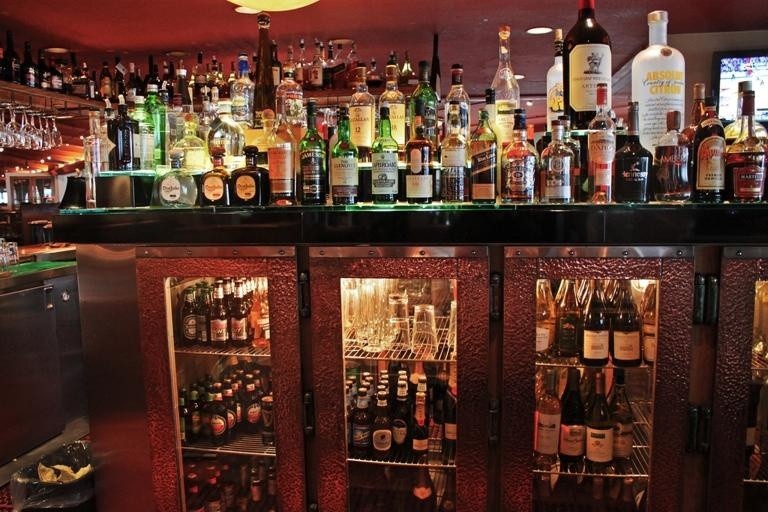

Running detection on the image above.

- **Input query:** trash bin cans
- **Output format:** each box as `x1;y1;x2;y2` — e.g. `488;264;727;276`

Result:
10;445;96;512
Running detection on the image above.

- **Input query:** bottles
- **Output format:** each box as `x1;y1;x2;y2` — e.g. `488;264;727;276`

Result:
743;280;768;483
1;0;768;208
176;276;276;512
345;353;458;512
534;275;658;512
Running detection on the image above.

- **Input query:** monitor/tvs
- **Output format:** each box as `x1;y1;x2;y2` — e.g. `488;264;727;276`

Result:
709;49;768;130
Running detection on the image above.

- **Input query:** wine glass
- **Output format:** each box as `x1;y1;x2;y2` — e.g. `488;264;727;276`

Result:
0;96;63;152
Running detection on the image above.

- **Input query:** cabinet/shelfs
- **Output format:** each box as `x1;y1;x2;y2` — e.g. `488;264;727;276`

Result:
309;246;491;512
705;246;768;512
134;245;308;512
499;245;696;512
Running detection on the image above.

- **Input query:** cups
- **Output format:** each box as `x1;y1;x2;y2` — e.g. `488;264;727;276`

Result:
340;278;457;357
0;237;21;279
